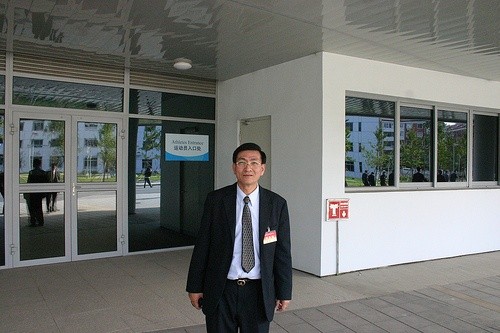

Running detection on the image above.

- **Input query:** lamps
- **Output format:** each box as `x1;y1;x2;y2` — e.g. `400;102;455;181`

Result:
172;57;192;69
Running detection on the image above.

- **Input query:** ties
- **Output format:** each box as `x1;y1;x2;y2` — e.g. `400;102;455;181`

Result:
241;196;255;273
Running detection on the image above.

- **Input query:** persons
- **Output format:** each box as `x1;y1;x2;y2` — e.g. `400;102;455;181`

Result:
444;170;450;182
362;170;370;186
411;166;428;182
0;164;4;214
379;171;387;186
23;157;49;226
367;172;375;186
185;142;293;333
143;166;153;188
46;162;61;212
388;170;395;186
438;169;445;182
450;169;458;182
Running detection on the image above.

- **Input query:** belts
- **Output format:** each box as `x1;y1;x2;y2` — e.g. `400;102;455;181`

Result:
226;278;261;286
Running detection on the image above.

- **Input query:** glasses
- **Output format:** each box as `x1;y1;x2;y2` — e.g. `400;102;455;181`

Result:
235;160;262;167
